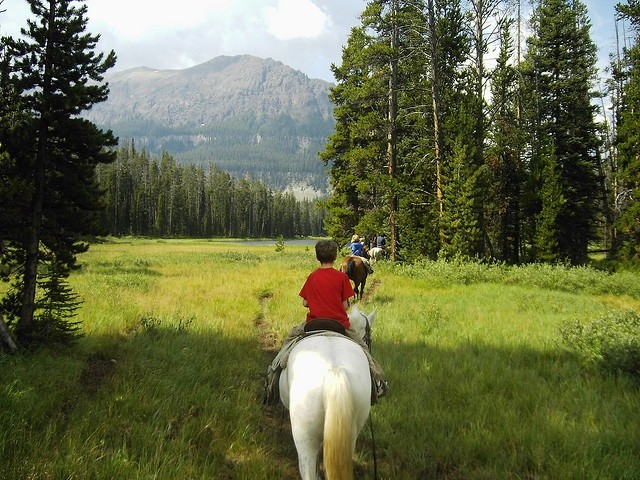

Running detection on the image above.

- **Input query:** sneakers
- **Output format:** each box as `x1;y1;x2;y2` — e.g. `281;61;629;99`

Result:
376;379;388;397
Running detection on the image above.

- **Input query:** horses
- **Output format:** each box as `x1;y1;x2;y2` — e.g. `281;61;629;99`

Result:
339;252;374;303
367;245;389;262
278;303;379;480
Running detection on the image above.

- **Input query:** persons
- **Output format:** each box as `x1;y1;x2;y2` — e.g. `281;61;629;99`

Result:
272;240;388;397
372;233;389;255
350;234;374;274
359;238;365;245
